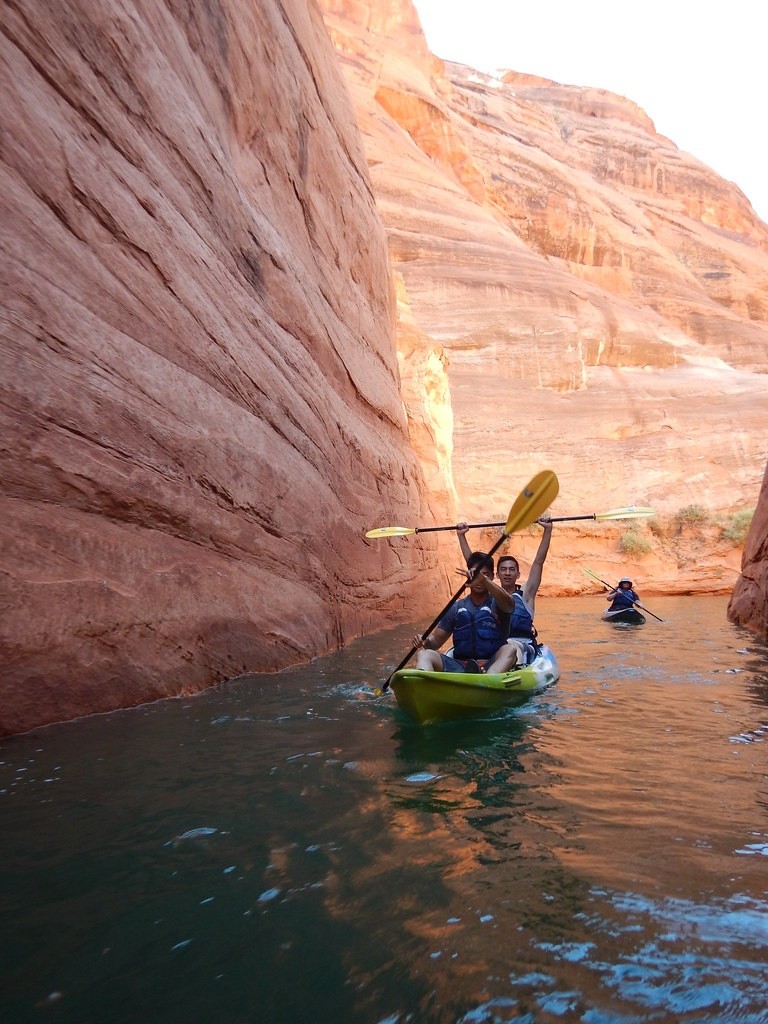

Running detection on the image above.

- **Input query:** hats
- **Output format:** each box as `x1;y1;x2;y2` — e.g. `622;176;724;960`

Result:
619;577;632;589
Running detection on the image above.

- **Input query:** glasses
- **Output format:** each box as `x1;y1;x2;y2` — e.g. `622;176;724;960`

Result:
623;581;631;584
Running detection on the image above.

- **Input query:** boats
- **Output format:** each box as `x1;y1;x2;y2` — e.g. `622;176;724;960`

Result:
389;644;560;727
602;608;647;623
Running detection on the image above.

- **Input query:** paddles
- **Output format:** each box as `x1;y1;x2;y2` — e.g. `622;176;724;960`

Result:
379;469;559;691
365;505;655;539
582;567;664;623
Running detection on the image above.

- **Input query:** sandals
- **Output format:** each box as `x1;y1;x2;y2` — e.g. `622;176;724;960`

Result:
464;659;486;674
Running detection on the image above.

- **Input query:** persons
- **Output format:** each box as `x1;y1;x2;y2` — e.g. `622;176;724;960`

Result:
606;576;641;611
457;515;553;670
411;551;518;674
